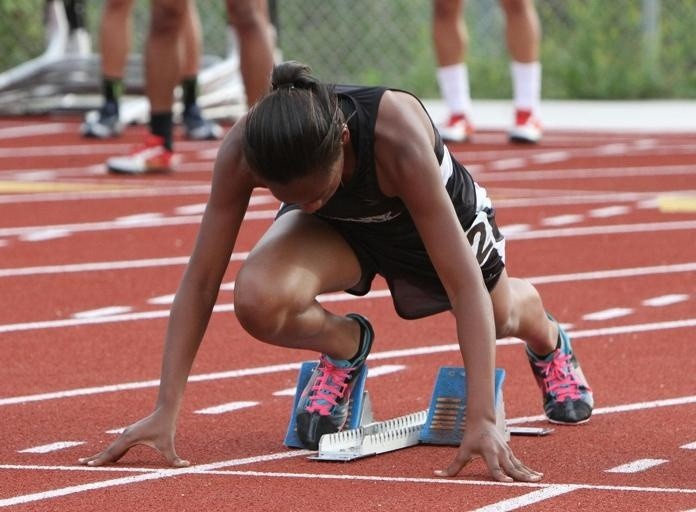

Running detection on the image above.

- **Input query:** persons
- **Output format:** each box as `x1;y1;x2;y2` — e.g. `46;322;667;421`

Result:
431;1;544;146
76;64;595;482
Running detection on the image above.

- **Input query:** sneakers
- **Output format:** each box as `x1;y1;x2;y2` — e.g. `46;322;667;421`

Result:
524;311;594;424
105;134;175;175
438;114;475;144
507;108;545;144
181;105;225;141
296;313;374;453
81;103;123;138
